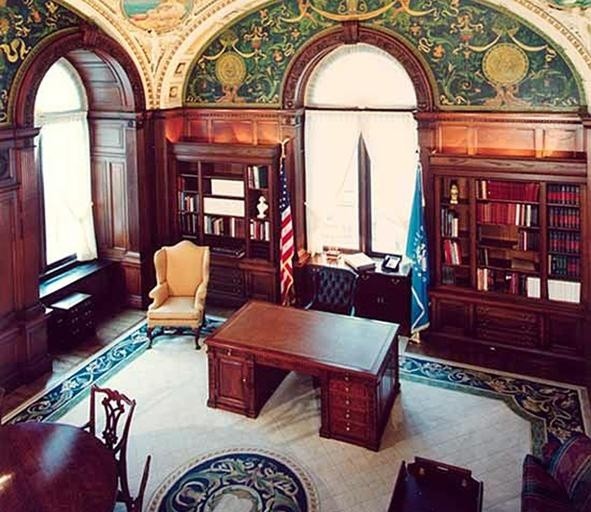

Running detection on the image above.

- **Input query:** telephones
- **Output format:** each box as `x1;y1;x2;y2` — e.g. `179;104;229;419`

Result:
382;255;401;270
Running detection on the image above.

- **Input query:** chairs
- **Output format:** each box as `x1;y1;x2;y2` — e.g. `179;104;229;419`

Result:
77;384;136;511
112;454;152;511
520;440;591;510
385;456;483;512
145;240;212;351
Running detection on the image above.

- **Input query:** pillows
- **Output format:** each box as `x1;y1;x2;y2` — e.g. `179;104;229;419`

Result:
551;429;591;510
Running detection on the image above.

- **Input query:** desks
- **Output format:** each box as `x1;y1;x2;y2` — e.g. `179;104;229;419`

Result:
0;420;120;512
51;292;97;349
202;296;402;453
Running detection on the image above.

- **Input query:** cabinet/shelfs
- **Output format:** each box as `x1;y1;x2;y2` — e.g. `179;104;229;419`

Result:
419;148;591;370
172;137;285;310
305;249;414;338
0;124;54;394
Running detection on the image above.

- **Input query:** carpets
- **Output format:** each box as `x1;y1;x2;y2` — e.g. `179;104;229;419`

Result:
1;315;590;512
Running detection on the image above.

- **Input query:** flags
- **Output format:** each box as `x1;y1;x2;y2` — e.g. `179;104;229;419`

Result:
278;152;296;308
404;168;433;334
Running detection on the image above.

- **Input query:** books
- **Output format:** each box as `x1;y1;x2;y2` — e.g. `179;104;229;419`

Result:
177;177;200;235
248;218;270;241
203;177;245;238
439;207;462;284
247;165;265;188
326;248;342;262
546;182;582;305
345;252;376;271
475;178;541;299
